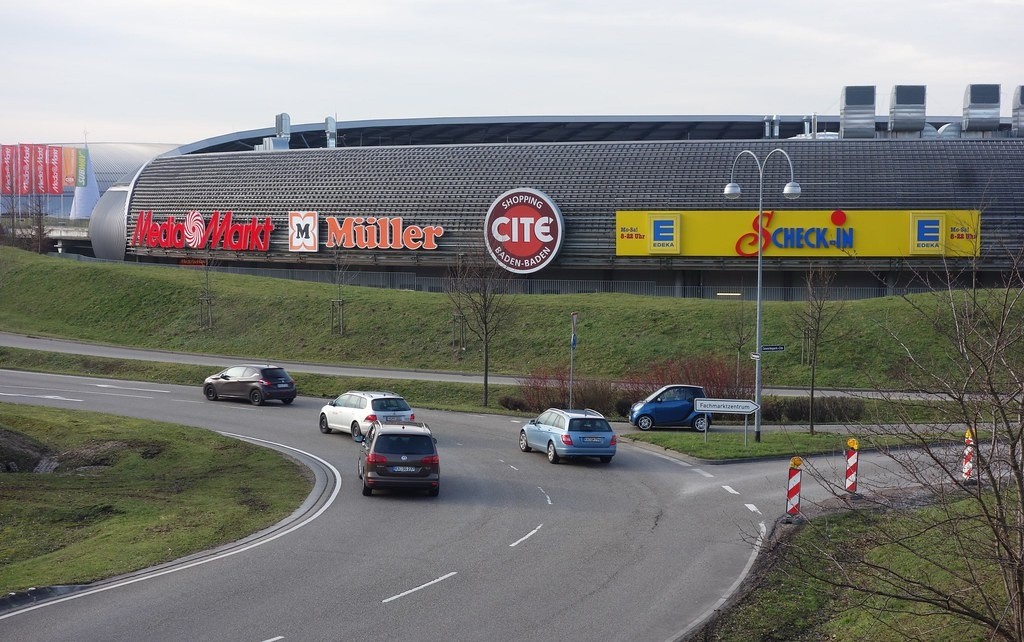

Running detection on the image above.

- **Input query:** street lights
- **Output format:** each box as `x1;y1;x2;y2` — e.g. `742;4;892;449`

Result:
726;146;802;441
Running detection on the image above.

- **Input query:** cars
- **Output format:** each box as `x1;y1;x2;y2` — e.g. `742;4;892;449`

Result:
203;364;299;405
628;384;713;434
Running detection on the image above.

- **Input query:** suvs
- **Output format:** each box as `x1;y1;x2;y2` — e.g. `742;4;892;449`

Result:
318;390;415;435
518;407;617;464
353;420;441;499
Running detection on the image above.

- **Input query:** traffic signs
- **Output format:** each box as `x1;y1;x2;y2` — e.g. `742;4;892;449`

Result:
696;398;761;416
